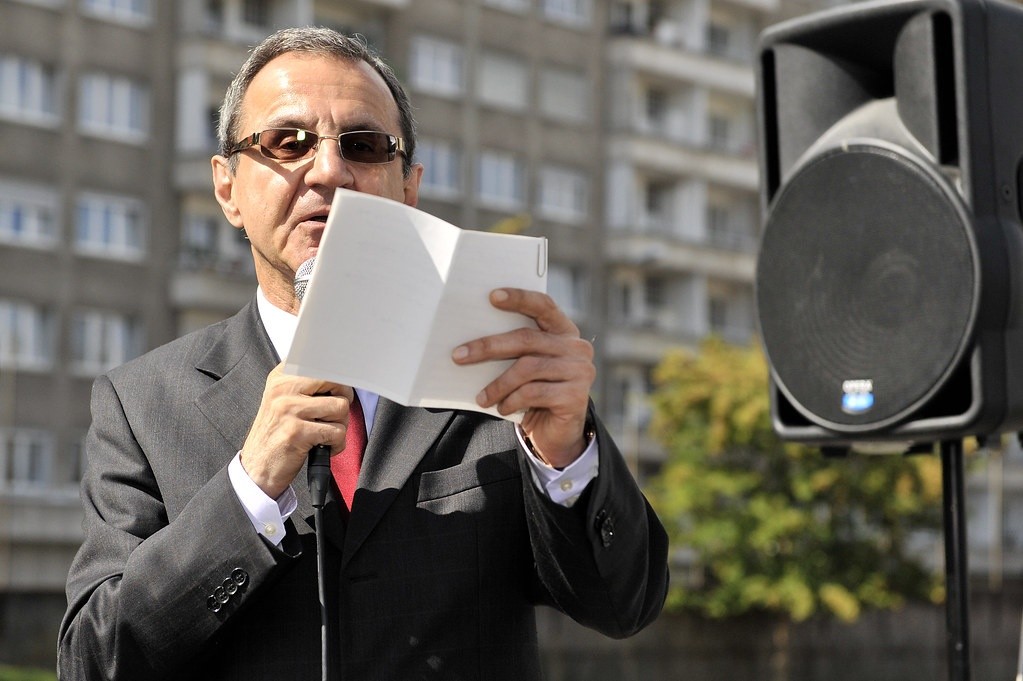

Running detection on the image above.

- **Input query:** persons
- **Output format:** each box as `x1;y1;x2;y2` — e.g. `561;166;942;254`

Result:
55;28;669;681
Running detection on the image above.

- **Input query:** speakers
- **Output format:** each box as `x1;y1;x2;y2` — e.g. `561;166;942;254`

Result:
754;0;1023;452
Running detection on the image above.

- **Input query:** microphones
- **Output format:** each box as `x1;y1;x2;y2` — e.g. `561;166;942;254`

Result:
292;256;331;508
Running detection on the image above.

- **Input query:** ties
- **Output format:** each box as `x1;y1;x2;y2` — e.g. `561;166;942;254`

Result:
329;387;369;511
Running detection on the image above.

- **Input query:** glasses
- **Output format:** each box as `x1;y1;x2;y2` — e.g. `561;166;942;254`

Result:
231;127;408;167
